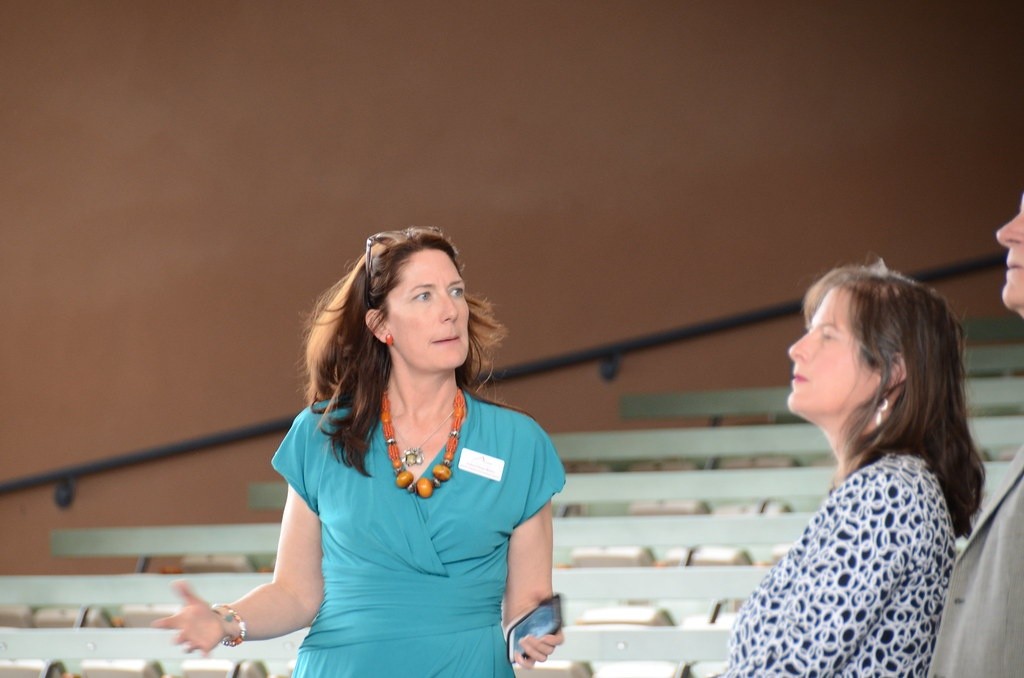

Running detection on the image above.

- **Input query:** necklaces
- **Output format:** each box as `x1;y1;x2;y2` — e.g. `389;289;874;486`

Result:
381;388;466;499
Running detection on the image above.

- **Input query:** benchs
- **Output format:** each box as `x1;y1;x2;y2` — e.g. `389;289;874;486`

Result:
0;314;1024;676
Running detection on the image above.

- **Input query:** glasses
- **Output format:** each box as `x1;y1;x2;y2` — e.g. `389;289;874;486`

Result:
365;225;443;308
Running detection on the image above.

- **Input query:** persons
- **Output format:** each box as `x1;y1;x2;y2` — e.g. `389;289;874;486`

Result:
708;258;983;678
155;225;568;678
931;184;1023;678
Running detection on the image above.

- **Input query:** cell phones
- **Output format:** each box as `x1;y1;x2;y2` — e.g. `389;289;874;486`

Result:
505;592;564;662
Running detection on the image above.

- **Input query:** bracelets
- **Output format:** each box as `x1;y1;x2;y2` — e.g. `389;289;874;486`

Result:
212;604;247;648
